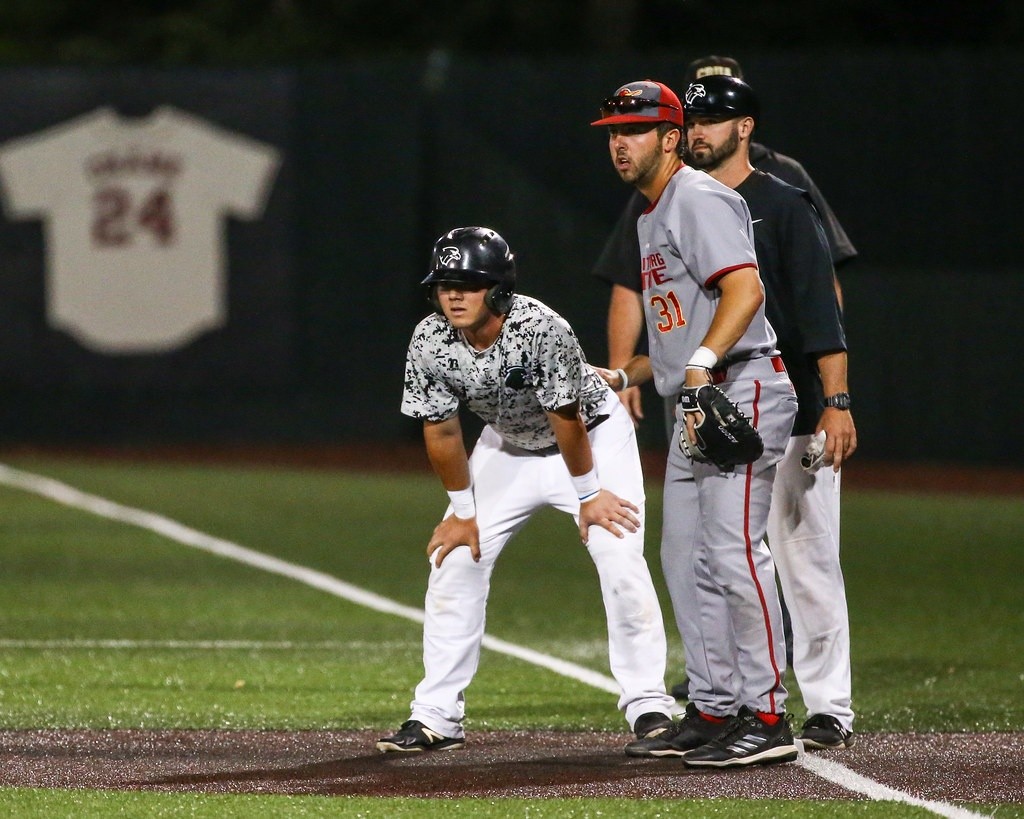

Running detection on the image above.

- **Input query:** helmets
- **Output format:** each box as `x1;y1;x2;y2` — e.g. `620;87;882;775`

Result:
678;74;758;122
420;227;516;315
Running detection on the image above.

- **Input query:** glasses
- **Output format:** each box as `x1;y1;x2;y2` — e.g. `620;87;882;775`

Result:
598;95;679;115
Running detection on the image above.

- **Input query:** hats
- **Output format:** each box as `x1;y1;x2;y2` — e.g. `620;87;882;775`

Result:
590;80;683;129
687;55;744;80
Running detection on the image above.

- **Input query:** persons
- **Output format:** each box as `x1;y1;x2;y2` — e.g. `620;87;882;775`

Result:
586;79;799;769
376;225;676;758
593;55;860;749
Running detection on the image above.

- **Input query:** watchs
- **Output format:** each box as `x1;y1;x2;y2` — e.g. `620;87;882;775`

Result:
822;390;852;411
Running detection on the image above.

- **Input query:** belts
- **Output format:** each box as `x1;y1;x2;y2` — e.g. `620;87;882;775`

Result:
517;415;609;457
709;356;784;385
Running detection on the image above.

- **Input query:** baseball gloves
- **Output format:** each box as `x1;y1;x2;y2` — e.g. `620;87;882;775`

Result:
679;383;765;472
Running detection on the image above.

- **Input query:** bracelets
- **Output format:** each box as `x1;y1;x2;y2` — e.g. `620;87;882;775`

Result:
685;345;718;371
615;369;628;393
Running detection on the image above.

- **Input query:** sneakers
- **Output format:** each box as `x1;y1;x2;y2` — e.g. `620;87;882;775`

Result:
633;712;673;739
671;677;693;700
798;714;856;749
682;704;798;768
377;721;465;752
625;703;736;758
